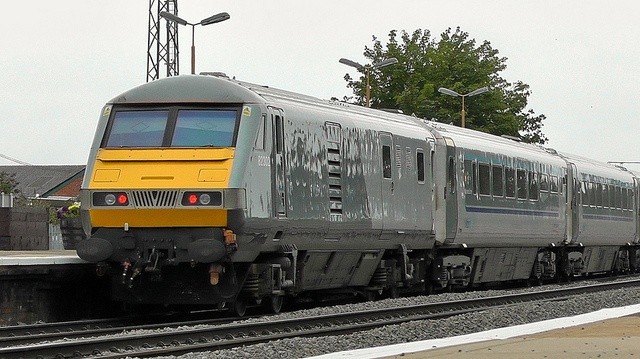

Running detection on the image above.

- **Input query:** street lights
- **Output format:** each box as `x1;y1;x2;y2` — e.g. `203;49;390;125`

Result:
158;11;230;73
438;87;493;126
339;58;400;109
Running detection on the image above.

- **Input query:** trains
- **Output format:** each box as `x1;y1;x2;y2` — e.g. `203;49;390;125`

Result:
75;72;639;317
